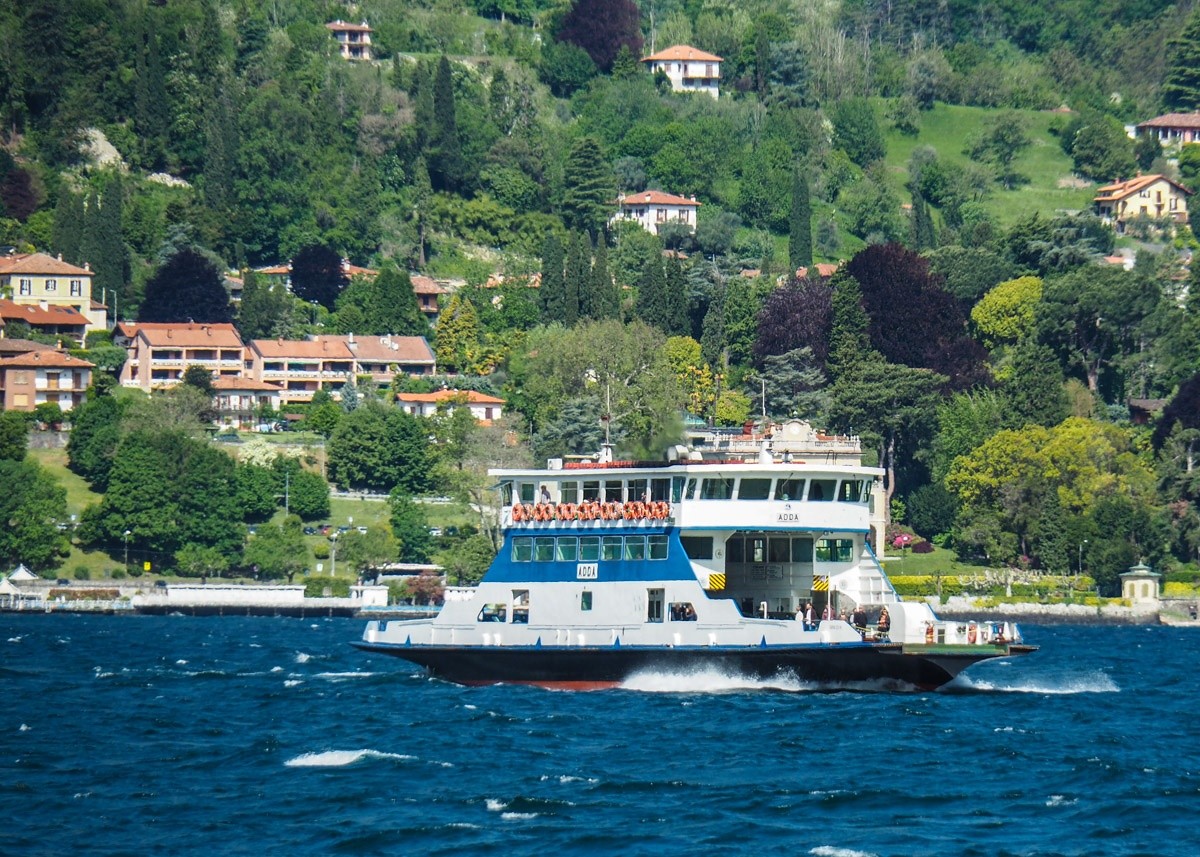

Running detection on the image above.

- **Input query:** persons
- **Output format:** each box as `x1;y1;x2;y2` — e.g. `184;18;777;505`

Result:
762;481;824;501
252;565;259;579
355;577;362;599
587;480;730;503
795;602;890;643
541;486;551;504
672;603;693;621
496;610;520;623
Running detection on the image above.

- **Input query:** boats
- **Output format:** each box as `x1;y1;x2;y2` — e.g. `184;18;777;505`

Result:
346;366;1041;696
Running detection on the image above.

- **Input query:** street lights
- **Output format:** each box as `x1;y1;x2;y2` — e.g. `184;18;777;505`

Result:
901;536;908;575
125;530;131;563
1078;539;1089;573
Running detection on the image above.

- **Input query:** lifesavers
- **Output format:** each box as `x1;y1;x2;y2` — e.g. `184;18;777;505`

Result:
600;501;622;521
577;501;599;521
623;501;645;521
646;501;670;521
556;503;576;521
512;503;534;522
533;503;555;522
968;625;977;643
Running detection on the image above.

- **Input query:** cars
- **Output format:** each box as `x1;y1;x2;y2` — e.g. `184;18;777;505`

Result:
303;526;317;536
429;524;478;540
327;524;368;542
318;524;332;536
